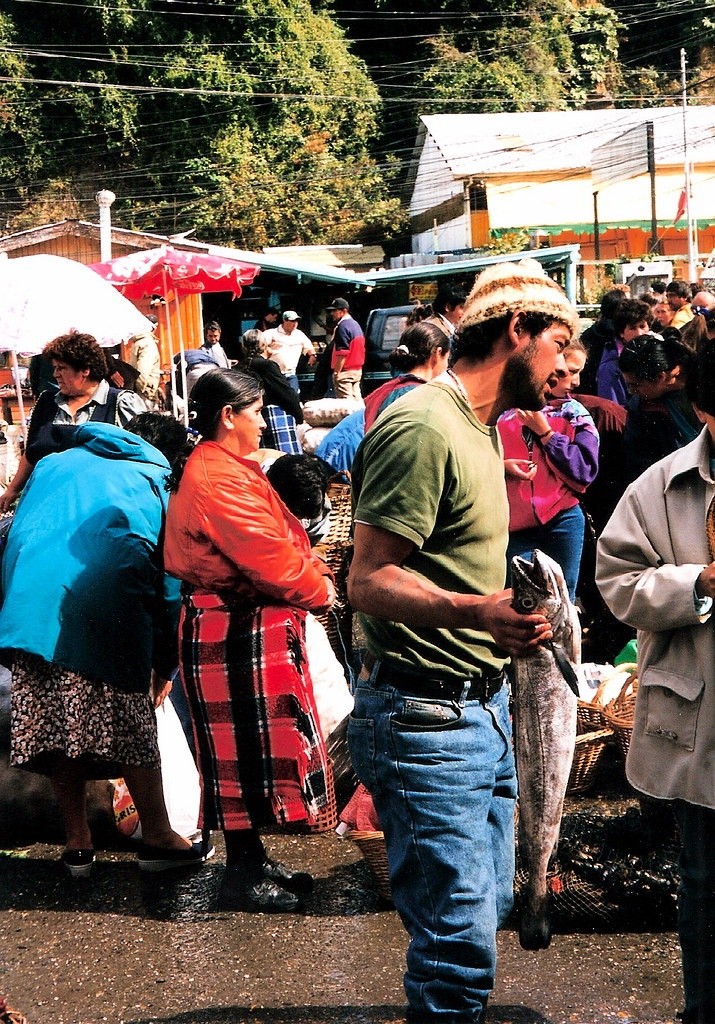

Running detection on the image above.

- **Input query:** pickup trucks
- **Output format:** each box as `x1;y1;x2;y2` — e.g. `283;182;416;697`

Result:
297;303;431;397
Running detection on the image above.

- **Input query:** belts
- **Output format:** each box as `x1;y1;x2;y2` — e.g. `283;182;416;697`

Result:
363;651;505;701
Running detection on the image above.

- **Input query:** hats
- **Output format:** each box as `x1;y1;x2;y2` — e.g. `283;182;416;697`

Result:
326;298;349;309
457;262;575;338
282;310;301;320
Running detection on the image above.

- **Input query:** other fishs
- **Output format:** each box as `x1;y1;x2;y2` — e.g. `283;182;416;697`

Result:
511;548;581;951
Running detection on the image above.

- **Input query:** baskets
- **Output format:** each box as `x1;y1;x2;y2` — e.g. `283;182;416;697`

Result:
346;827;392;899
315;471;354;659
567;661;639;792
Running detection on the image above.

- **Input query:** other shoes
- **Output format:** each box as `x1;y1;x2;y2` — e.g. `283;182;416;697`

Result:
63;849;93;878
246;857;314;893
218;878;299;910
136;841;215;871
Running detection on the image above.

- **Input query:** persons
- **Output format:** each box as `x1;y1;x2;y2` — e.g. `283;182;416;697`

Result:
132;315;159;410
0;334;147;512
346;258;579;1024
578;282;715;642
165;370;336;906
170;293;467;540
0;411;203;860
596;336;715;1024
497;343;598;600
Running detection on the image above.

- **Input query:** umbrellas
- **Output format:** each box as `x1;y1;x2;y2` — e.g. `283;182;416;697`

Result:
87;246;260;423
0;254;157;444
